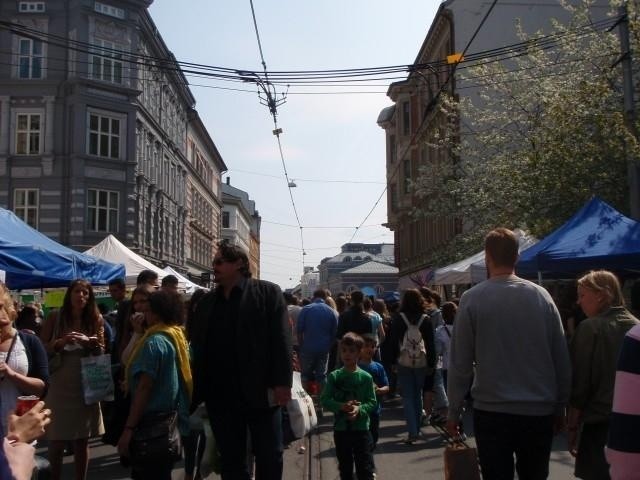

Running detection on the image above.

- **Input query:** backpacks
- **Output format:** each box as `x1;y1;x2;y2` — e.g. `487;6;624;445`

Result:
397;312;428;368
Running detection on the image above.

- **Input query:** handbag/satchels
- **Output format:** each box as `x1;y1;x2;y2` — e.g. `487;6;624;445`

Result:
287;371;317;439
121;413;182;465
444;432;480;480
80;352;115;405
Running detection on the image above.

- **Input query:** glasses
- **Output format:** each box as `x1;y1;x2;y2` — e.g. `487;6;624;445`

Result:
212;258;231;266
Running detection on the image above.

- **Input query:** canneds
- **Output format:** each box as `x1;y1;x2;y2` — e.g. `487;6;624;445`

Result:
16;395;39;416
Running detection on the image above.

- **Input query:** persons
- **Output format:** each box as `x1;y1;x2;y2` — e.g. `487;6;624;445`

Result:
604;324;639;480
565;270;639;480
